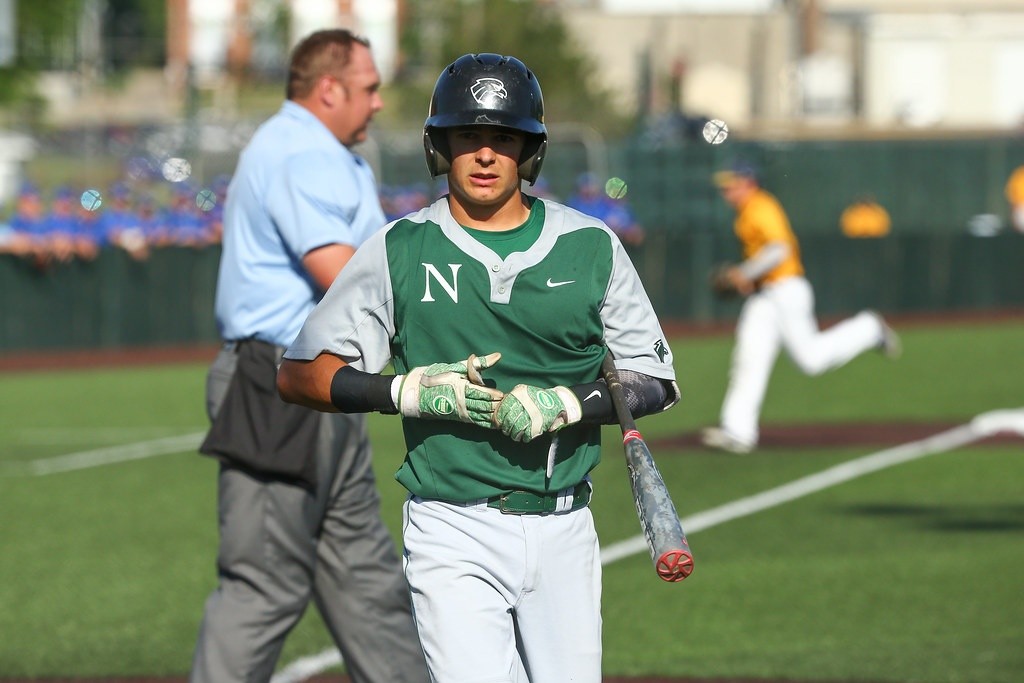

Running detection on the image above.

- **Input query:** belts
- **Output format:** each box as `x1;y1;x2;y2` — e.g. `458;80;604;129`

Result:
221;337;242;353
487;479;591;515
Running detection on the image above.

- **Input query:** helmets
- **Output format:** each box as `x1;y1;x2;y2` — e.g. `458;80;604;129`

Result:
423;52;549;188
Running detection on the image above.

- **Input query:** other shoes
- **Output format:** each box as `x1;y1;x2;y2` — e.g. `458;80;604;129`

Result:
703;426;753;457
871;311;901;362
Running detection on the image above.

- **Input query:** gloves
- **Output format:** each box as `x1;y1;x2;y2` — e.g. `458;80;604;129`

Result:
389;352;500;430
493;384;582;444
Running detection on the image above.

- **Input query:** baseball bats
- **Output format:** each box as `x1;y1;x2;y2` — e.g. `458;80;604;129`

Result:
602;351;694;583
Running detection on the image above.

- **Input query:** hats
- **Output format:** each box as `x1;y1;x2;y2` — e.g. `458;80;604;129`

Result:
709;160;757;187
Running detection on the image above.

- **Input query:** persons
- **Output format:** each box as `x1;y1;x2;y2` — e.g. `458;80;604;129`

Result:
277;50;683;683
696;151;902;453
188;28;430;683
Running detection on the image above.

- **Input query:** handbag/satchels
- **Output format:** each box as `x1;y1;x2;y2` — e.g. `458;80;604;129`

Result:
198;340;320;494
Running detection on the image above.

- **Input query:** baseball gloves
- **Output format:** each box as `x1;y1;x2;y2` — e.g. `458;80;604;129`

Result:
711;259;736;298
